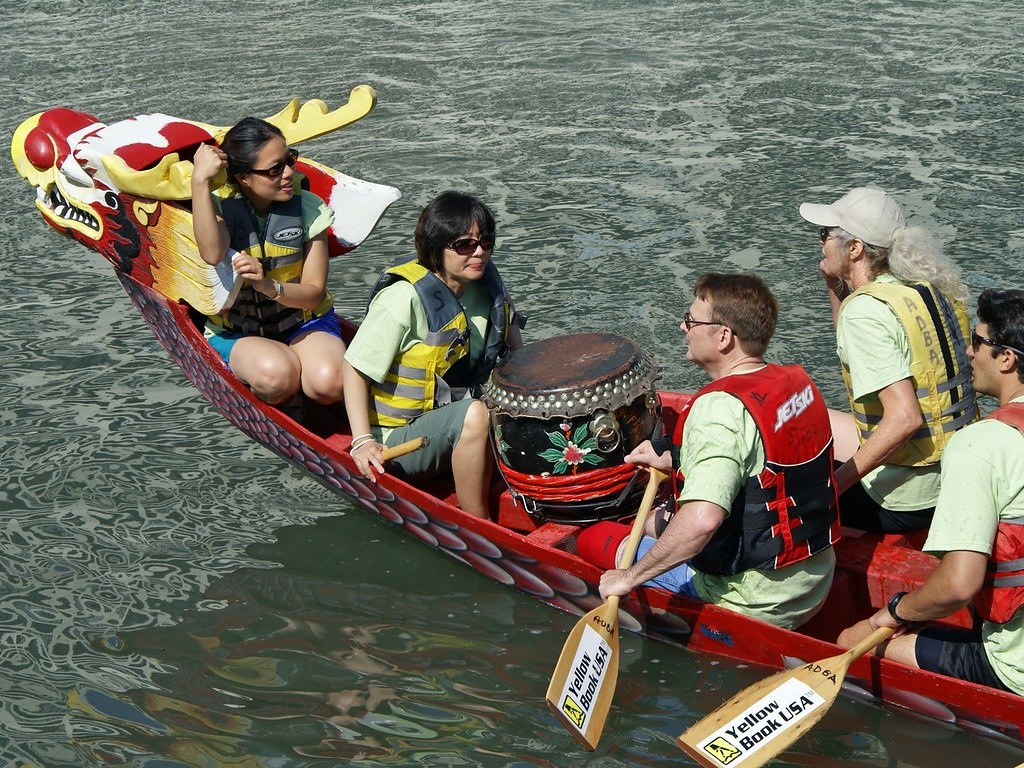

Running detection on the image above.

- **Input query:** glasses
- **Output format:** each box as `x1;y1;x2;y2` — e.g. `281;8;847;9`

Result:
972;329;1024;357
445;233;494;255
820;227;876;251
684;312;736;336
241;147;299;177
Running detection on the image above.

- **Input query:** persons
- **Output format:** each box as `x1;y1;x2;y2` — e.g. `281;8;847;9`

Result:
598;270;842;633
799;186;984;536
342;190;528;524
193;117;345;404
837;288;1024;697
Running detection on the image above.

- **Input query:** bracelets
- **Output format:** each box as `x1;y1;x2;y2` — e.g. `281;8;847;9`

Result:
350;434;375;455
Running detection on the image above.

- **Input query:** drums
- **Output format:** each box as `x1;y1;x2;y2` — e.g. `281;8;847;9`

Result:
480;329;675;532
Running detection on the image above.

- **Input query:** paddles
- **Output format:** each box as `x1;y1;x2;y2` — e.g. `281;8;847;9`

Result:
668;621;908;768
543;463;678;754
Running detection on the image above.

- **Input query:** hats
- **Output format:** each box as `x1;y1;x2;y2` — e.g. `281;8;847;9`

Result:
799;187;906;248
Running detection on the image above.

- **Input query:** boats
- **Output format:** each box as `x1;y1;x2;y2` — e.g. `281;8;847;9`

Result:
10;83;1024;753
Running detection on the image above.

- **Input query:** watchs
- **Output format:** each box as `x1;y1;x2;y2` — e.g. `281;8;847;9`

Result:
266;279;285;301
888;592;912;624
